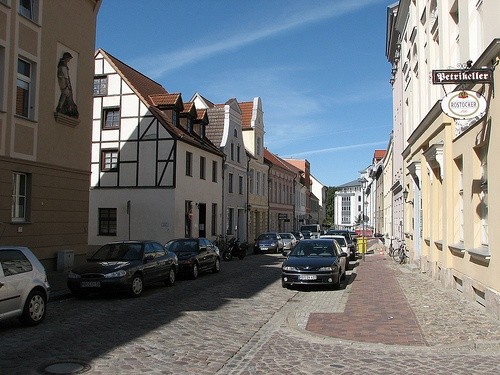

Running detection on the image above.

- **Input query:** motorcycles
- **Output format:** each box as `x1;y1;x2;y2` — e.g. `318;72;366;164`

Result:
212;234;249;262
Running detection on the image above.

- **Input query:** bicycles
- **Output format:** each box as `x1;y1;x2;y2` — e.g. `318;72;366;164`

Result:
387;237;409;264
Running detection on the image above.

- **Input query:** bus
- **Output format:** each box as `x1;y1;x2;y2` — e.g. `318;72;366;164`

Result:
301;224;321;240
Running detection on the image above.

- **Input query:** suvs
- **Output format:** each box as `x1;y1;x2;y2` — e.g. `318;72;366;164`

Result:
327;229;356;261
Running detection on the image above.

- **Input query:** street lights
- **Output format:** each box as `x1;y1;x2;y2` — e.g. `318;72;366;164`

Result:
359;176;369;261
365;201;369;230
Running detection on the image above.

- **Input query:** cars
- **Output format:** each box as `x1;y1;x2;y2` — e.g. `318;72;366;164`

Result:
0;246;50;328
319;235;354;271
301;230;315;239
291;231;305;246
278;232;296;251
254;232;284;254
67;239;179;300
282;239;348;290
164;237;220;280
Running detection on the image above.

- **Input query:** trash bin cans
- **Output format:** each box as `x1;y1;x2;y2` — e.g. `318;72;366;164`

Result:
356;238;368;253
57;250;75;269
